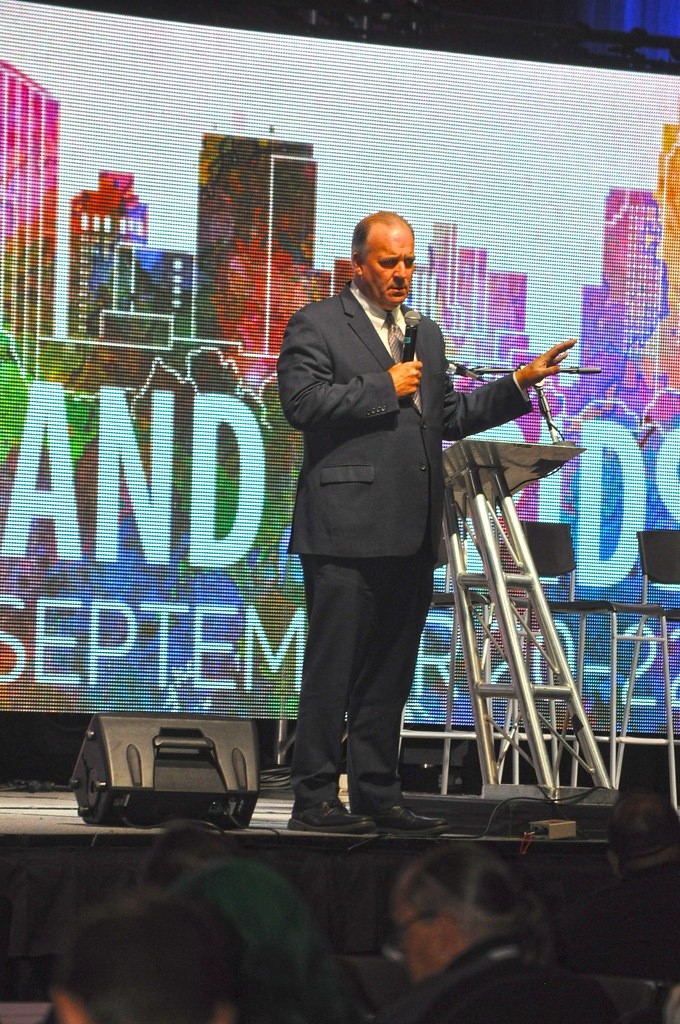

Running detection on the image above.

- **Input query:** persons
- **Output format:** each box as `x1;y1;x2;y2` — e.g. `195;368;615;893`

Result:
43;790;680;1024
276;213;578;833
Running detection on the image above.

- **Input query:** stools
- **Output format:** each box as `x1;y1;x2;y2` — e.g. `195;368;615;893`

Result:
615;528;680;790
511;519;678;811
398;528;533;796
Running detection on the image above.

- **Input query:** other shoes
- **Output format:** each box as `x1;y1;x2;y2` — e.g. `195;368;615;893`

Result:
368;805;449;836
288;800;376;832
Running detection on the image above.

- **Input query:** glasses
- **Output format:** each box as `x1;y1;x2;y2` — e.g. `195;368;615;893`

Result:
382;910;439;962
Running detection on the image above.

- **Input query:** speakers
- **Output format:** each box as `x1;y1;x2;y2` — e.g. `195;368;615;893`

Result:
67;713;260;832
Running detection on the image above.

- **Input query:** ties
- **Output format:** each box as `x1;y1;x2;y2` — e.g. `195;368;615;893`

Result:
384;314;422;414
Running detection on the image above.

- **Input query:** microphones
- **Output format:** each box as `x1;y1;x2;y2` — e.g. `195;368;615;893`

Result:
446;363;484;384
401;312;421;363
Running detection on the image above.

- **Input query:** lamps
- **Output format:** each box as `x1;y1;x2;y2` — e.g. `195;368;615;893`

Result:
68;711;261;830
